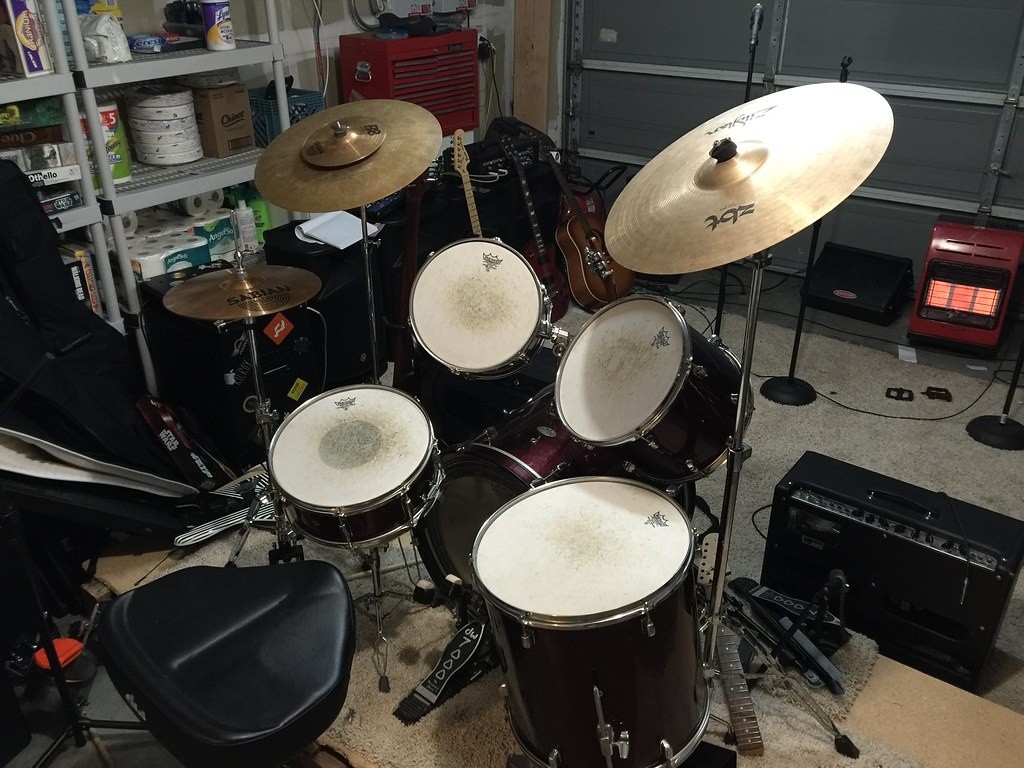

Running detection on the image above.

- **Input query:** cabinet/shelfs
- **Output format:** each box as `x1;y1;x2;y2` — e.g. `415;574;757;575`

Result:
0;0;126;335
60;0;313;398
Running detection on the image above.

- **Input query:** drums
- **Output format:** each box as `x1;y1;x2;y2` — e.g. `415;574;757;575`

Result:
266;383;443;552
408;236;553;382
469;476;712;768
412;380;699;607
552;292;757;484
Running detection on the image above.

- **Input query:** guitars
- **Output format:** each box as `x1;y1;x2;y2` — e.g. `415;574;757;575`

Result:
539;142;634;309
449;128;485;241
497;130;570;323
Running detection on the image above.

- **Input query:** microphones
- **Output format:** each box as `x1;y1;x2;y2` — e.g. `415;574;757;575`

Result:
821;569;852;593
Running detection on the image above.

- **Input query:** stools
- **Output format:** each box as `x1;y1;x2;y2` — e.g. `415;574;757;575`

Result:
101;562;356;768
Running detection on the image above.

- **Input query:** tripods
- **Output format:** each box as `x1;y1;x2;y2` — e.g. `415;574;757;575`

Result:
0;500;151;768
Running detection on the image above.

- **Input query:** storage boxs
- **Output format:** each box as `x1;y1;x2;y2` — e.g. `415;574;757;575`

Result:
192;83;256;159
248;86;324;149
0;0;55;79
23;164;82;187
0;140;76;171
40;190;83;215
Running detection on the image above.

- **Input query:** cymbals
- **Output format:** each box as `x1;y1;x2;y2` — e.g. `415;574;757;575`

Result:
162;265;323;321
254;97;442;214
604;82;897;276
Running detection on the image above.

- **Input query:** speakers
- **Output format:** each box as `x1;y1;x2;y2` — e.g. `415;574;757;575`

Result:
445;136;565;272
760;449;1024;695
142;222;388;480
800;241;914;327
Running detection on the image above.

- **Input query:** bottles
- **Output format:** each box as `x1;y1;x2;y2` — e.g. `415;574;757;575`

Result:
248;192;271;242
201;0;236;50
234;199;259;254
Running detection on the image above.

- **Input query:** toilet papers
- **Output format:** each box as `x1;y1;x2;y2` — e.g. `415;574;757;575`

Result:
85;209;139;241
159;188;225;216
76;205;240;281
